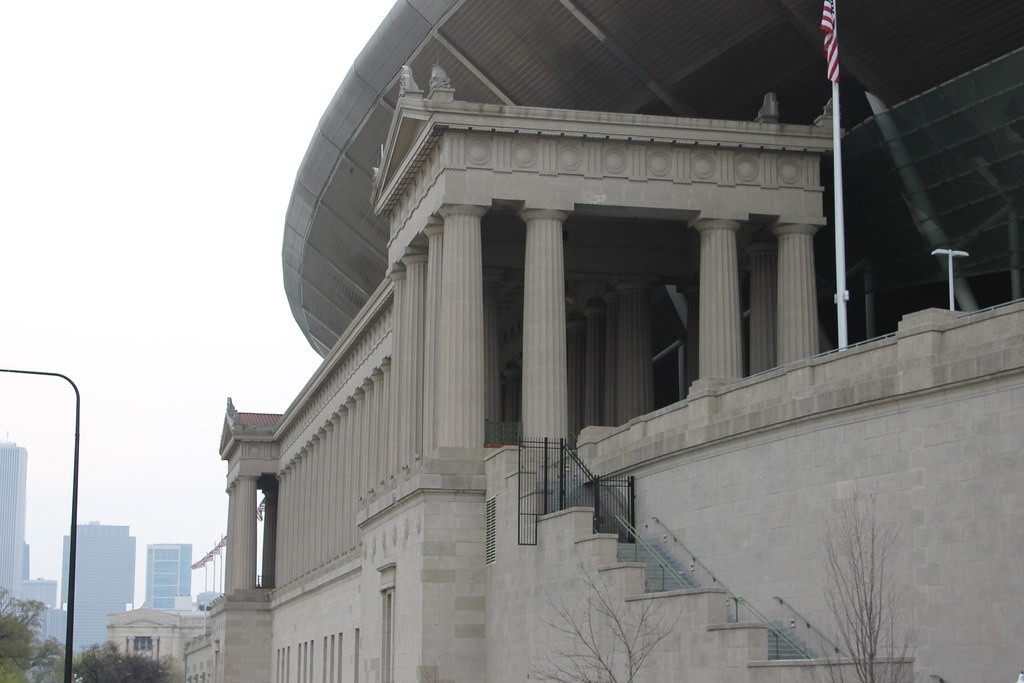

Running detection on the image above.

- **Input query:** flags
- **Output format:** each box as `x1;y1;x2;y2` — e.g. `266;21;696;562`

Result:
257;508;263;521
209;548;221;555
191;559;205;569
217;536;227;547
205;554;213;561
821;0;841;83
259;497;266;511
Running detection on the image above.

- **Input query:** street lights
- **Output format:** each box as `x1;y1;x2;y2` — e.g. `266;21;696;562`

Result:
931;247;970;312
